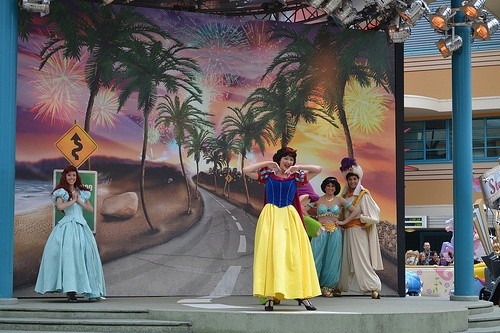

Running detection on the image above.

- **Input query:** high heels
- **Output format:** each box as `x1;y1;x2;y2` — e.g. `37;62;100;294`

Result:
265;300;274;311
298;299;317;310
66;292;78;303
89;296;106;302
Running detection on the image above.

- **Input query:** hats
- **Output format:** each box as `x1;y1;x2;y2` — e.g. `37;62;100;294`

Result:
339;158;365;196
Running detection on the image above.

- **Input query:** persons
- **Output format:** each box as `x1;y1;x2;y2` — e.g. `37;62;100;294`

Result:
405;241;454;266
310;177;359;298
339;158;381;298
34;165;106;301
242;147;323;311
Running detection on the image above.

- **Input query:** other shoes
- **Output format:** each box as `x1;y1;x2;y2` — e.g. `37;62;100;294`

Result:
372;292;380;300
322;290;334;298
333;291;341;297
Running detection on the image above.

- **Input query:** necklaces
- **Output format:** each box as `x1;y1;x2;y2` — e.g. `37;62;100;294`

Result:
324;195;335;201
